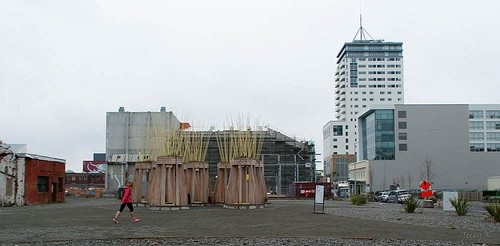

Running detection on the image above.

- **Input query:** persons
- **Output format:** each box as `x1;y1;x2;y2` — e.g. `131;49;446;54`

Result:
112;182;140;224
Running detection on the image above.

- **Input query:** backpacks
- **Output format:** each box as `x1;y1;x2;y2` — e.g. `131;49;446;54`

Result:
117;187;129;200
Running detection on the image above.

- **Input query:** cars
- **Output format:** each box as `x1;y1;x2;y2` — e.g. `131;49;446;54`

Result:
386;190;412;204
374;192;389;202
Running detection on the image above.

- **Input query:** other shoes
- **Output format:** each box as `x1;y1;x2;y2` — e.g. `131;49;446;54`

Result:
112;218;120;224
132;217;140;222
